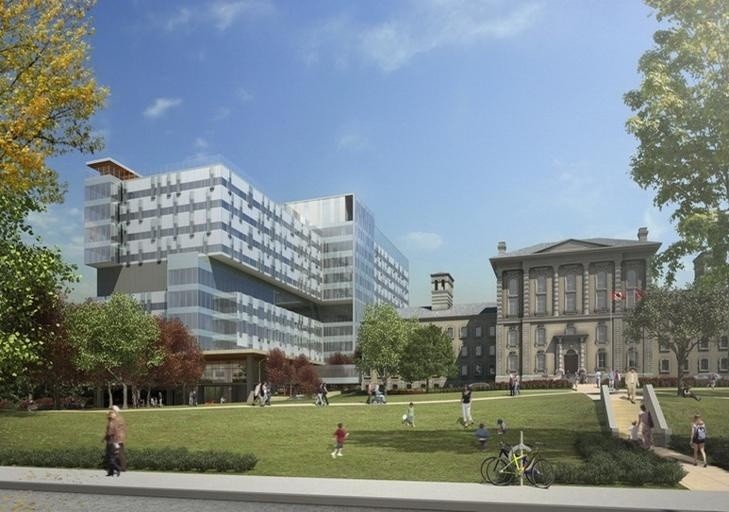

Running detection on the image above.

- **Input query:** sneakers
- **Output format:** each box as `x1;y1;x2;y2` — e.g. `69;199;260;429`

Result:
331;453;336;460
337;454;343;457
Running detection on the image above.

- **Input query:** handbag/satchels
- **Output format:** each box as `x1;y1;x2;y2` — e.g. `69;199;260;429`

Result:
648;412;653;427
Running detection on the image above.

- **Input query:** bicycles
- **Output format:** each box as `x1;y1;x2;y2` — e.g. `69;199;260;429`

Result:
481;442;556;488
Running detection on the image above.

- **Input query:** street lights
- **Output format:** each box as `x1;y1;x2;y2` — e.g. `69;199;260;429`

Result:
258;357;269;382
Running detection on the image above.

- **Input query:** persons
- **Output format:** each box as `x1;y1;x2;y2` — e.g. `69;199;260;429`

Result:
624;366;639;405
506;368;521;396
691;415;708;467
564;365;622;390
188;390;198;406
130;389;163;408
331;423;349;458
460;384;474;426
406;403;416;428
316;383;330;405
496;419;507;434
711;373;718;390
638;403;653;449
248;380;271;406
476;423;490;450
629;420;639;441
366;383;386;404
683;384;691;397
102;406;126;476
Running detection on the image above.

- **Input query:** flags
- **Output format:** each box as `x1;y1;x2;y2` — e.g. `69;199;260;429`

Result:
609;290;625;301
635;291;641;301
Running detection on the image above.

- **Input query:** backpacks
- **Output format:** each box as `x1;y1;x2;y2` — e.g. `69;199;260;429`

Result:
693;425;706;444
